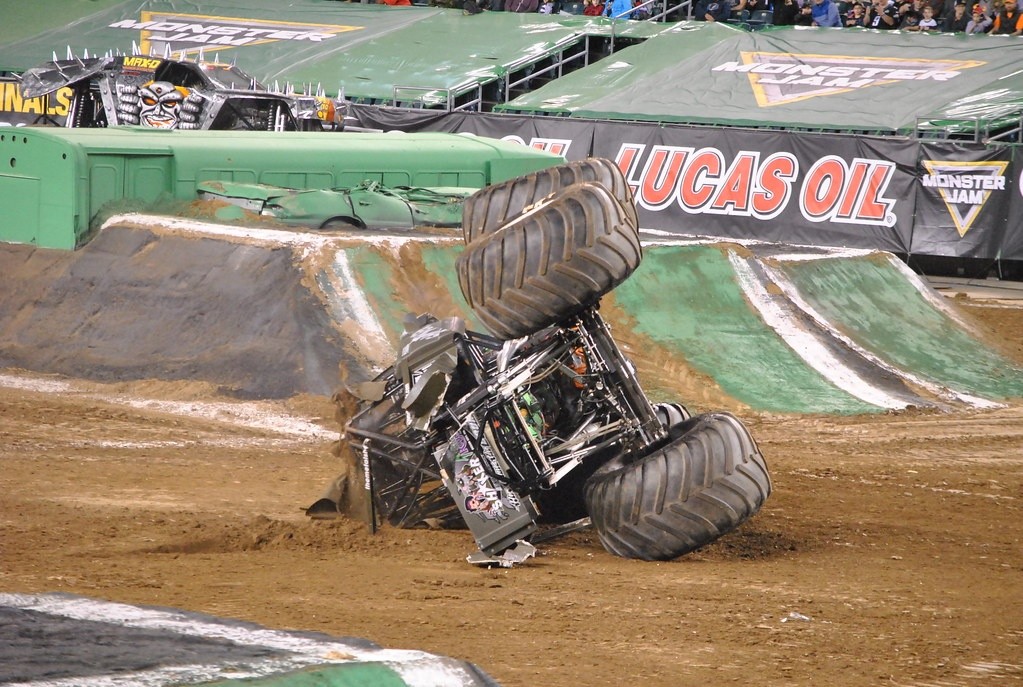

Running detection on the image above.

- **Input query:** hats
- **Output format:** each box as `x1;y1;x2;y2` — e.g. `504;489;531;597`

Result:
972;8;982;14
1005;0;1017;4
954;0;967;7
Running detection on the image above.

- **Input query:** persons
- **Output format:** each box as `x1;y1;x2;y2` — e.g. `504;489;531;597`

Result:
374;0;1023;37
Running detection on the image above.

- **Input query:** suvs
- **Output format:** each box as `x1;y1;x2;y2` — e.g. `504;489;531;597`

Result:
327;156;775;563
